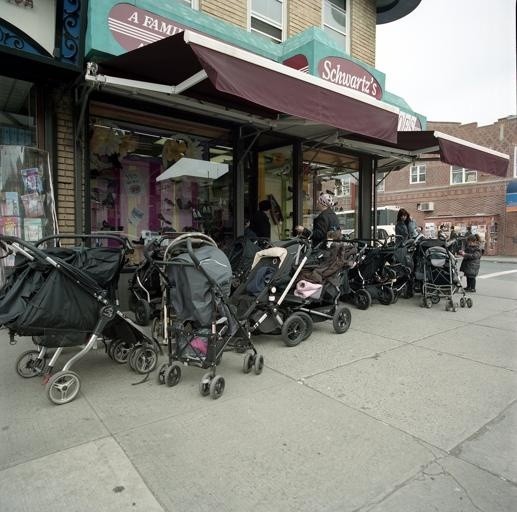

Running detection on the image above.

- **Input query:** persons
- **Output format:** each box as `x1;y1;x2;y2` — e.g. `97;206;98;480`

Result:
438;225;445;242
394;207;417;250
464;227;472;246
458;238;480;292
295;194;343;252
474;234;483;250
244;200;271;241
446;226;457;253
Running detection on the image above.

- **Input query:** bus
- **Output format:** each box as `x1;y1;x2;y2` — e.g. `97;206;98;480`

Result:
334;205;399;247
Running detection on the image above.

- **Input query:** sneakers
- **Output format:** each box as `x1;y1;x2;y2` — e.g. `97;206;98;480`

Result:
463;287;475;292
